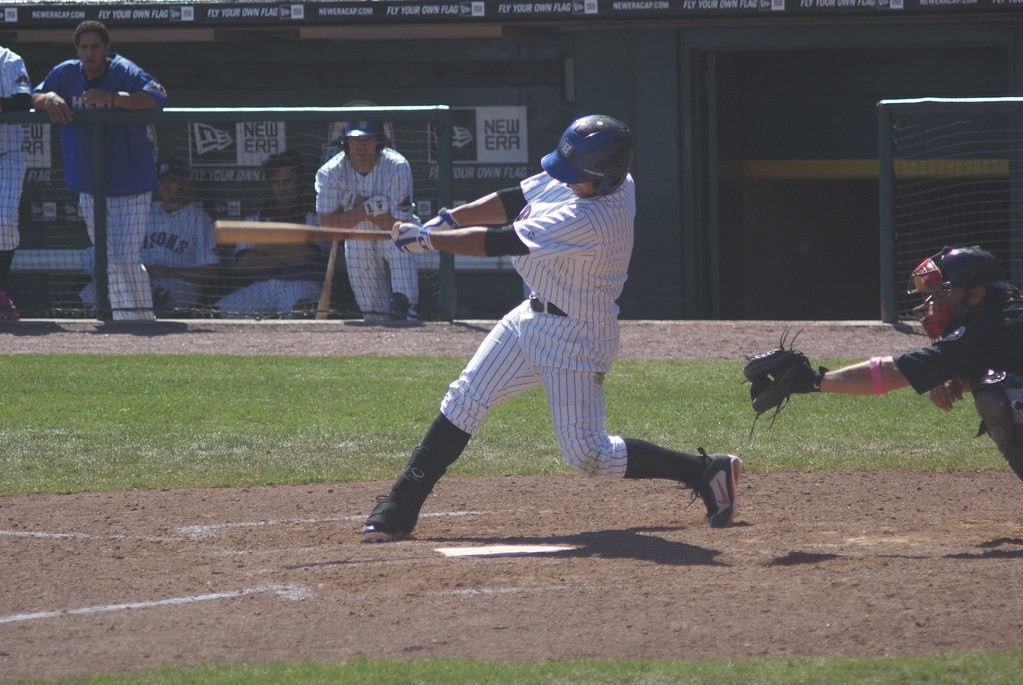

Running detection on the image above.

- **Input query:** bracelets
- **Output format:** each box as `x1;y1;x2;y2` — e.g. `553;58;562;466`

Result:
111;90;115;105
869;356;887;394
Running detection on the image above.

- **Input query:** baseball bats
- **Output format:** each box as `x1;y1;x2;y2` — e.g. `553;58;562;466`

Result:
213;218;394;249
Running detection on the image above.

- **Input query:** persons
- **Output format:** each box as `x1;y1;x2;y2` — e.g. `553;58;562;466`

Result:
31;20;167;319
744;244;1023;478
140;162;222;318
215;149;326;313
361;114;743;542
314;122;418;322
0;45;31;319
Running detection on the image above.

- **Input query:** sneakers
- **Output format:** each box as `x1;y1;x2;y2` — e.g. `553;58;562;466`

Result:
362;495;417;543
686;448;740;528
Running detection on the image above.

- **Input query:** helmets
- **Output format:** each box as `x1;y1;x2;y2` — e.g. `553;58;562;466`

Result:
341;119;384;136
541;114;633;196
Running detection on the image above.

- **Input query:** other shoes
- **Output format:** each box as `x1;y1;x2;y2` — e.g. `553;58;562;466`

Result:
0;292;19;319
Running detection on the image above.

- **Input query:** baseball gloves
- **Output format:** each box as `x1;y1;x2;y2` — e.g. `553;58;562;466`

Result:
738;323;829;443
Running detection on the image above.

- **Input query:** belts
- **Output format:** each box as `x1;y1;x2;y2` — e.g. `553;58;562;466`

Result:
529;294;567;318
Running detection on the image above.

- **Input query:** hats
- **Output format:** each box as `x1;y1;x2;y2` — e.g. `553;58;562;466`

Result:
931;246;1018;291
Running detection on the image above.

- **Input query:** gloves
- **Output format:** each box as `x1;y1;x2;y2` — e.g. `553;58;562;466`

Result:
364;195;390;217
422;207;458;233
391;221;435;255
